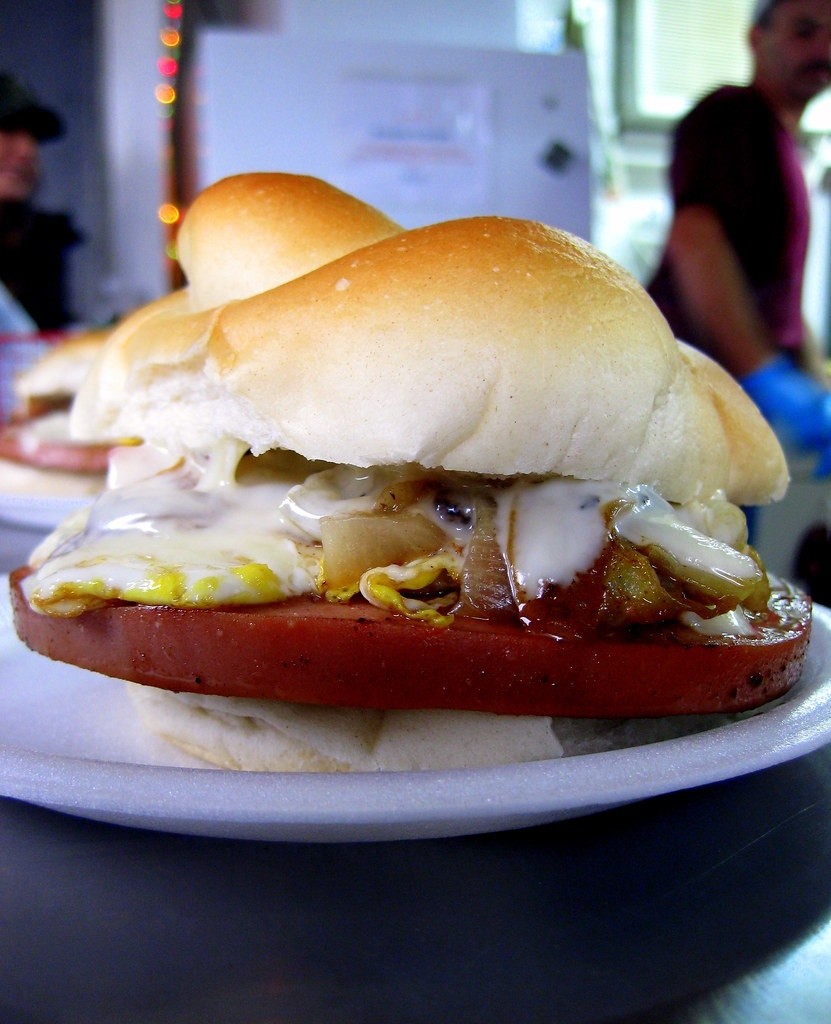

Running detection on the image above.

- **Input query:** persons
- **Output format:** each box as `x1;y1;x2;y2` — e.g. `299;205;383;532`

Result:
646;0;831;548
0;72;86;330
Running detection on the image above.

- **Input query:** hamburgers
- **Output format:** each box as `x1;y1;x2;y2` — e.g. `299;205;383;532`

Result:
0;172;811;773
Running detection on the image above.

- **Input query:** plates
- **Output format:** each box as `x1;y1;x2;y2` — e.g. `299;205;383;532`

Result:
0;578;831;846
